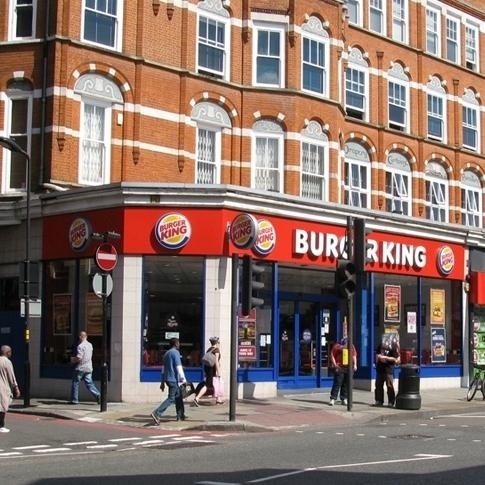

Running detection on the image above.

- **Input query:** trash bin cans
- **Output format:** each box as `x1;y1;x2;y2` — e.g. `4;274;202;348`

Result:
395;363;422;410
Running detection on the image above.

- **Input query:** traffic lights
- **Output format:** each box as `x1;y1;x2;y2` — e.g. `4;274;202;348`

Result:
334;257;358;300
353;217;374;273
241;255;266;317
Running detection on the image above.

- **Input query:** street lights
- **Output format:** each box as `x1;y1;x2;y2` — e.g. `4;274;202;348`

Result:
0;137;31;407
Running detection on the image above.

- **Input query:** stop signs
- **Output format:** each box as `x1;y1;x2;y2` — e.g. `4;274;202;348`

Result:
96;242;118;271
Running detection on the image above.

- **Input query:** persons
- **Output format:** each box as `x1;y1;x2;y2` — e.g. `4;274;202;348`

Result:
0;345;20;433
193;336;225;407
329;337;357;405
372;336;401;407
151;338;187;426
67;331;101;405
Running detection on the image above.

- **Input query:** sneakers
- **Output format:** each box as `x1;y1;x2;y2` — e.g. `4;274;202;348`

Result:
194;399;199;407
329;399;347;405
151;413;160;425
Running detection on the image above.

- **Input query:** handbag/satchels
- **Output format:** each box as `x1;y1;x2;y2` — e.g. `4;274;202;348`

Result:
202;347;216;367
196;375;225;398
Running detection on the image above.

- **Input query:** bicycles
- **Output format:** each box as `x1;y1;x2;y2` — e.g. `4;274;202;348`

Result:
466;362;485;401
